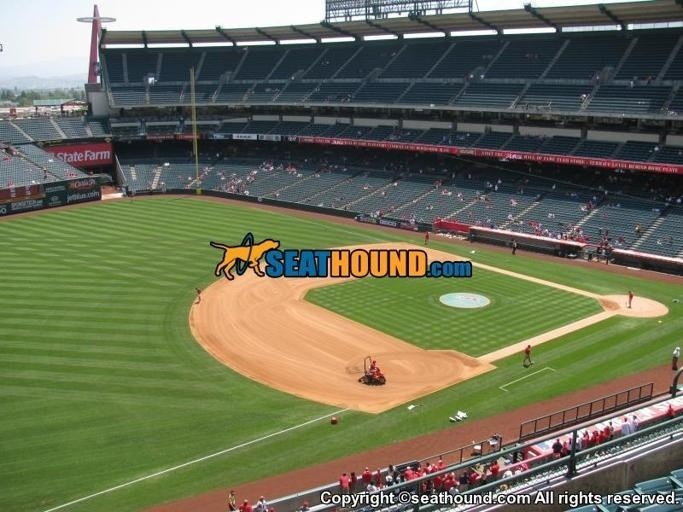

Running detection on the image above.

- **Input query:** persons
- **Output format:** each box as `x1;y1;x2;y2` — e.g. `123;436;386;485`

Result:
226;401;675;512
523;345;536;367
369;360;381;381
627;290;635;310
157;145;682;266
672;345;680;371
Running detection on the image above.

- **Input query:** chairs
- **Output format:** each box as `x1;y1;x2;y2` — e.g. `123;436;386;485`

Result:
566;468;683;512
